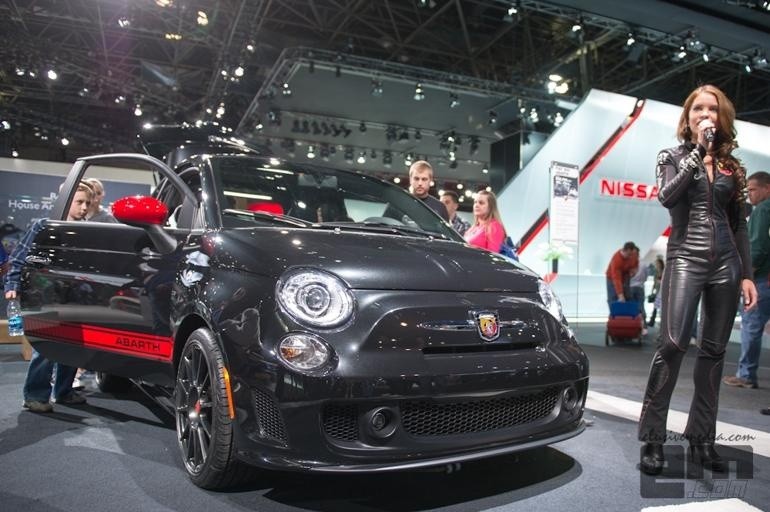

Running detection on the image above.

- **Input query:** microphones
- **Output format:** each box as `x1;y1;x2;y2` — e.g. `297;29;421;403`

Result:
696;119;715;145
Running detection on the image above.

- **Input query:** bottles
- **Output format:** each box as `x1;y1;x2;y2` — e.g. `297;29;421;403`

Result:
6;297;23;337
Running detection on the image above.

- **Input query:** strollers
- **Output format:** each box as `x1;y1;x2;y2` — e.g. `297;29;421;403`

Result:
604;282;648;347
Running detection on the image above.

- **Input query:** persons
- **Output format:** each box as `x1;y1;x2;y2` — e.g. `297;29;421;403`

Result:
645;260;664;327
462;188;506;253
3;182;101;412
605;240;639;317
722;170;770;390
68;176;116;390
397;158;450;231
637;85;762;478
440;191;471;240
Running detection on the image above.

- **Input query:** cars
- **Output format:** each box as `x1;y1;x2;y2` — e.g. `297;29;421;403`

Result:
18;122;590;491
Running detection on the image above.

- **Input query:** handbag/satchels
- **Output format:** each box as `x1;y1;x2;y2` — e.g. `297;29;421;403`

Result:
648;294;655;303
499;236;519;262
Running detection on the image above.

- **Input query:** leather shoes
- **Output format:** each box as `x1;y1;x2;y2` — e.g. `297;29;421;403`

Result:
640;441;665;476
688;442;725;472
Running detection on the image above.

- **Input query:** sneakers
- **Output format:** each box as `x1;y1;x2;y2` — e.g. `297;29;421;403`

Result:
22;398;53;413
722;375;758;388
55;391;87;405
